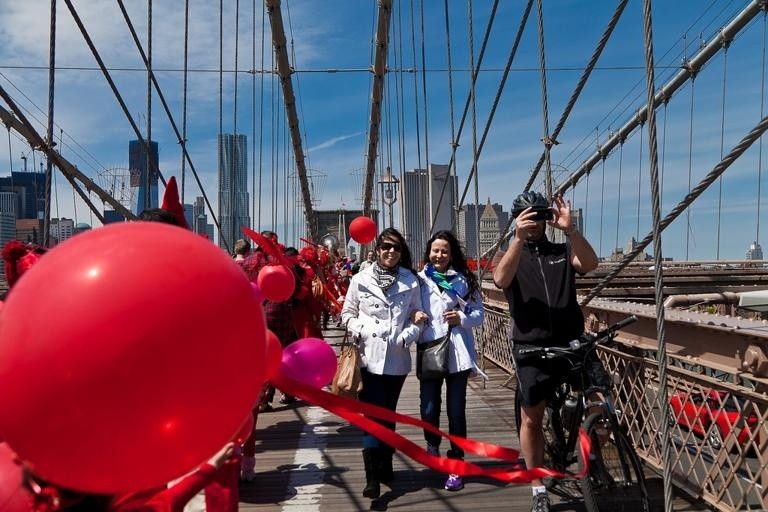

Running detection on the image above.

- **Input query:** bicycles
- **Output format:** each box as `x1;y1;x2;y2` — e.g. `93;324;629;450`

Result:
515;315;652;511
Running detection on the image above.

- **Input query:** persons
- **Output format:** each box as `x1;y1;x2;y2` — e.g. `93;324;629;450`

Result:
340;227;424;501
19;443;235;512
411;229;485;492
136;189;373;475
493;191;610;512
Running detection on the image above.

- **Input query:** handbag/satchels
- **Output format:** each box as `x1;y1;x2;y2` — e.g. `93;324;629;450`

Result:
415;336;451;380
332;345;363;399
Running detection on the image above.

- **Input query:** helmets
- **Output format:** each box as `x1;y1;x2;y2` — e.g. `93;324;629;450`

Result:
511;190;548;218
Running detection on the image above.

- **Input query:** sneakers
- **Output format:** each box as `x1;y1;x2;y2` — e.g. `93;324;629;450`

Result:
443;460;465;492
426;440;441;461
530;486;551;512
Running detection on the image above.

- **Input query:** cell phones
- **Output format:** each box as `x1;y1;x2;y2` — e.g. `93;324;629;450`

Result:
529;208;553;221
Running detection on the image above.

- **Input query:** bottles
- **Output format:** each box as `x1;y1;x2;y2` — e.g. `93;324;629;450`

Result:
561;394;580;431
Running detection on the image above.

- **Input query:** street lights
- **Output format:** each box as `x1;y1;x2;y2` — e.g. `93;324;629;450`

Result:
378;166;400;228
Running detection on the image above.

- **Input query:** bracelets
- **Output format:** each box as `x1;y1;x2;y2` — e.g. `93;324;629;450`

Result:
564;223;578;237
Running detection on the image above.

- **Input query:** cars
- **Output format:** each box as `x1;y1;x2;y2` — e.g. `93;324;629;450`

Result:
647;265;673;274
667;389;765;449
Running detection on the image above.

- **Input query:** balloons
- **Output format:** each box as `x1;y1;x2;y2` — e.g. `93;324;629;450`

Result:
0;220;267;494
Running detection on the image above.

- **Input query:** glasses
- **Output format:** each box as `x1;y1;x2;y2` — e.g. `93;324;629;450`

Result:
381;243;402;251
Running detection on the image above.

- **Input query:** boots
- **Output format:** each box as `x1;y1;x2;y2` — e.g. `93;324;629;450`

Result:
362;443;395;498
